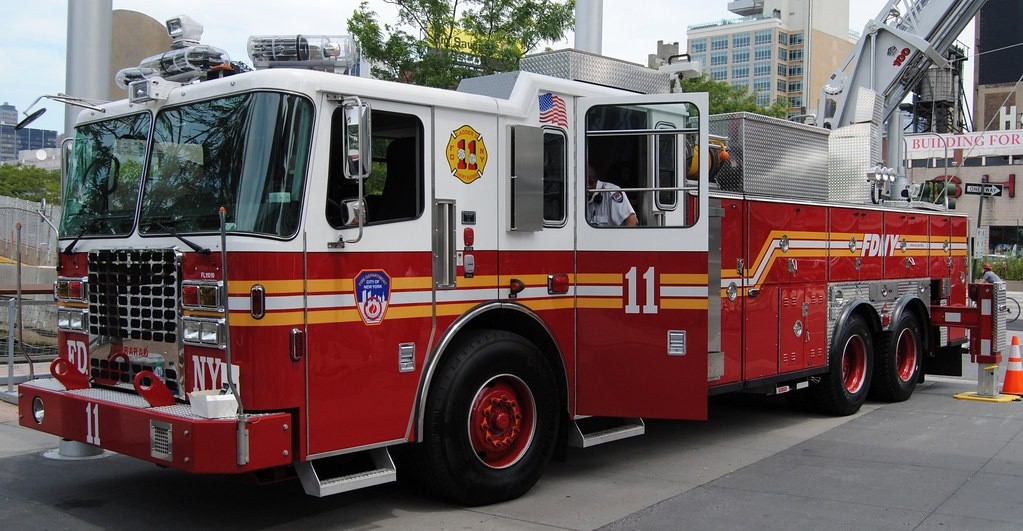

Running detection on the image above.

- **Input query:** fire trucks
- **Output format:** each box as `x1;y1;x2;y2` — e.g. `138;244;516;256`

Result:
13;0;1007;511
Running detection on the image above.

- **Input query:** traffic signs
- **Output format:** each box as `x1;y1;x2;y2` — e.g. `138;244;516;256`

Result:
964;182;1003;198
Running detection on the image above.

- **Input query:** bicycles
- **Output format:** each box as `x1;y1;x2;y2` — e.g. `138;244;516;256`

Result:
1006;296;1021;324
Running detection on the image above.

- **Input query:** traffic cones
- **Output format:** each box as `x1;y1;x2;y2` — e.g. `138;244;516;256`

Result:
998;335;1023;396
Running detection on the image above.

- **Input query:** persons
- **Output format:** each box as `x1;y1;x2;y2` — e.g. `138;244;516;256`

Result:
585;167;638;227
979;263;1003;284
